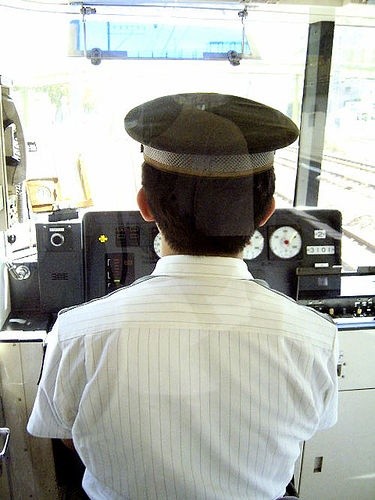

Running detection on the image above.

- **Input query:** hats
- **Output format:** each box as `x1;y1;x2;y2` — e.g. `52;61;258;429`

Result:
123;93;306;183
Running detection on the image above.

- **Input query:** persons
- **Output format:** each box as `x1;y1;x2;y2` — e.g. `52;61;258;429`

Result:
24;90;339;498
